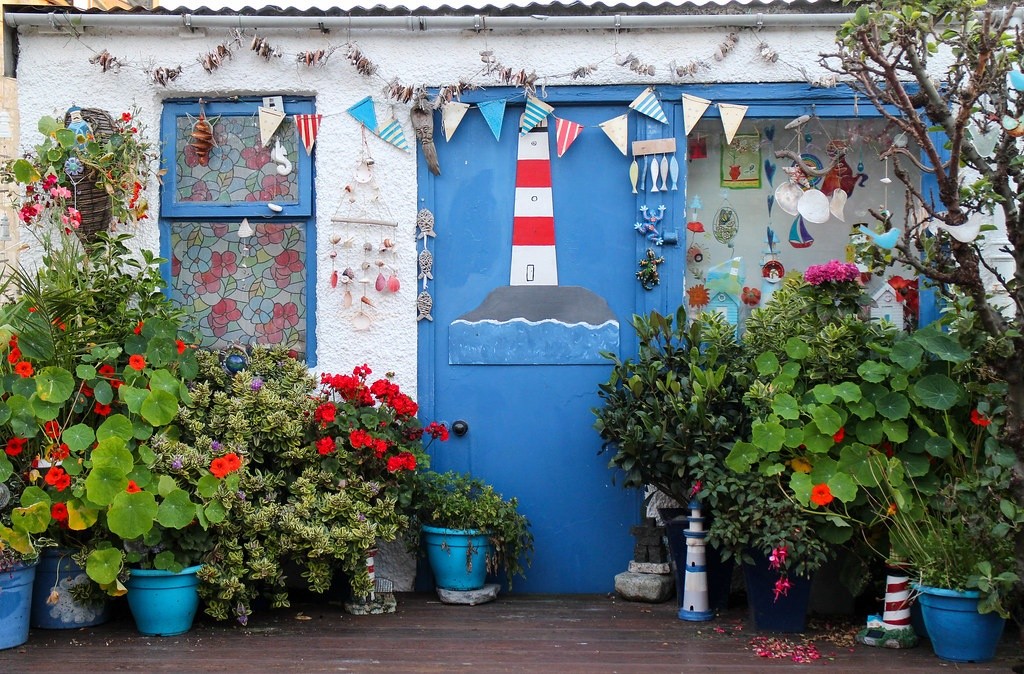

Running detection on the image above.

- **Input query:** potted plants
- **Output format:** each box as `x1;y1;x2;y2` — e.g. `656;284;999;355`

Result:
416;470;536;592
852;456;1012;663
587;305;755;610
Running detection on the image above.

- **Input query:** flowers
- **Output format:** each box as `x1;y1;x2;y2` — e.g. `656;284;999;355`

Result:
0;97;449;628
689;259;1024;619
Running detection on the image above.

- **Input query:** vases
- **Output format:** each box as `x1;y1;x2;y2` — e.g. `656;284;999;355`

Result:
0;560;37;650
739;545;815;633
29;549;117;631
122;564;201;637
